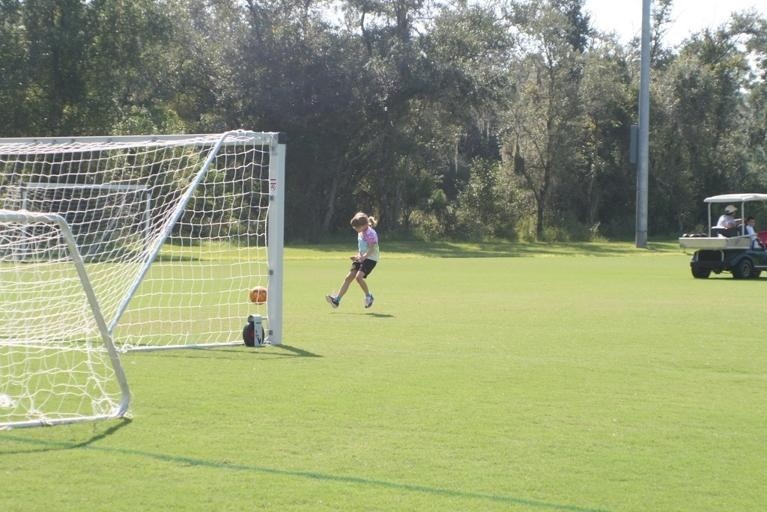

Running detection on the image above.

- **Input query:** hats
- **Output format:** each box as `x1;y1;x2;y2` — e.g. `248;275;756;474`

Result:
724;204;738;215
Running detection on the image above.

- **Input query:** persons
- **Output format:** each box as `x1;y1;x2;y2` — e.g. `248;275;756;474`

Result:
323;209;379;309
744;216;763;249
716;204;741;238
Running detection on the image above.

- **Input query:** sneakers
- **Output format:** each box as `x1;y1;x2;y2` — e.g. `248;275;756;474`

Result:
364;294;374;309
325;295;339;309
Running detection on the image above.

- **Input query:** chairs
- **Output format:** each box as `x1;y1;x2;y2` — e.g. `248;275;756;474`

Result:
711;227;738;237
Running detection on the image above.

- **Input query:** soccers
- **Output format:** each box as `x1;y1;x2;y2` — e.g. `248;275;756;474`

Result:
249;286;267;305
